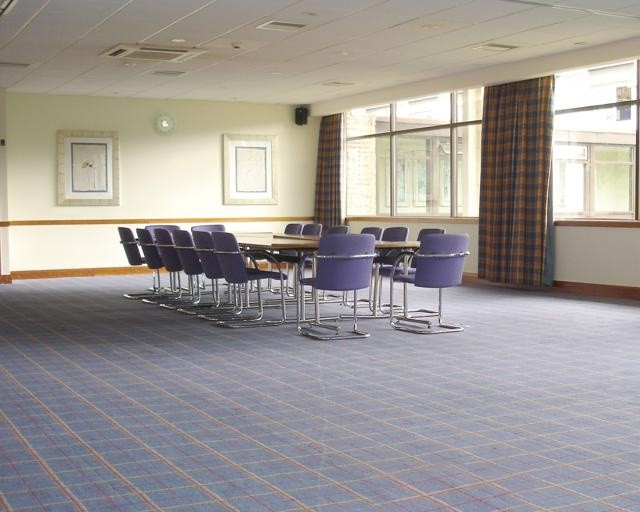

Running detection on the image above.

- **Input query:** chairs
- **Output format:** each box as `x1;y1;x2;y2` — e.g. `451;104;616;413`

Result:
298;232;376;341
388;233;470;337
267;222;447;319
114;223;290;331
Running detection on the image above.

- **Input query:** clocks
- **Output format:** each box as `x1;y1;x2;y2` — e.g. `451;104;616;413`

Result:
153;111;177;138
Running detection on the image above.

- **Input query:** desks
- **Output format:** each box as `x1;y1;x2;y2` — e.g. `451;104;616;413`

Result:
231;232;422;325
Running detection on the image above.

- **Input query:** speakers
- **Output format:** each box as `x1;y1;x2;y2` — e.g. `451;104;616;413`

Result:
295;108;307;126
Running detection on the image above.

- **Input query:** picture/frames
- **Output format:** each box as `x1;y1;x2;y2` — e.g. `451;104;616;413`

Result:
221;132;280;207
54;126;121;207
